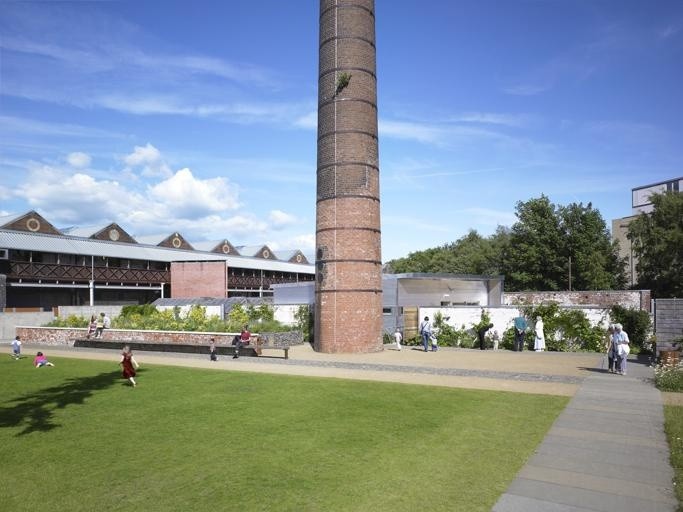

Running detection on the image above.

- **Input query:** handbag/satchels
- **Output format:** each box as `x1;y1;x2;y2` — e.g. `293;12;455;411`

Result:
421;327;423;334
232;336;240;345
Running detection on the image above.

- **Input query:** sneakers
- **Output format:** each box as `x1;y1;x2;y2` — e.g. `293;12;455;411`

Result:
608;369;626;376
233;352;239;358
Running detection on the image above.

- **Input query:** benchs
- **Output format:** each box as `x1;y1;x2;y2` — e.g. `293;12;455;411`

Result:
70;338;290;360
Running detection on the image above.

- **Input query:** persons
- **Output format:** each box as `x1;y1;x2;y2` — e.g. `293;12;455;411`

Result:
231;323;251;359
478;323;493;350
208;338;219;361
511;312;528;352
418;316;431;352
84;314;97;339
428;334;438;351
393;328;402;350
612;322;629;375
32;351;54;367
116;344;140;387
91;312;110;339
8;335;22;359
533;316;545;351
603;324;617;373
